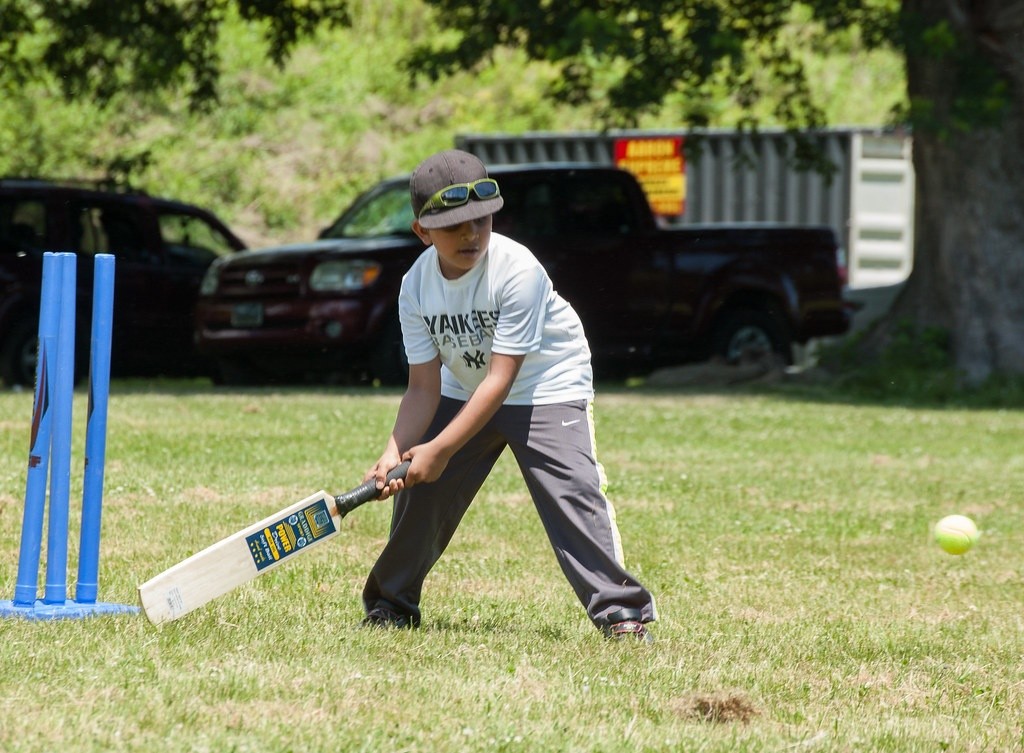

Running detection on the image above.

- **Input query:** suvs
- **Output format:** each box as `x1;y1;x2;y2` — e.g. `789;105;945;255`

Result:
0;176;249;385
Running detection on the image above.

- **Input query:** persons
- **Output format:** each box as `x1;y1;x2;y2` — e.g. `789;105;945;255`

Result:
362;150;657;642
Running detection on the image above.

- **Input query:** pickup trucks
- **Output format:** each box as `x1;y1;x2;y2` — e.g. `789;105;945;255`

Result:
197;161;851;397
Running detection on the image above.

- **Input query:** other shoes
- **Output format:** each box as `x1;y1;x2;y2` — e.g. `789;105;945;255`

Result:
603;618;655;650
361;608;408;632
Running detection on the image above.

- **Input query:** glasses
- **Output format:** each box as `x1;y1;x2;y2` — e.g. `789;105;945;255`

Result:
418;178;501;217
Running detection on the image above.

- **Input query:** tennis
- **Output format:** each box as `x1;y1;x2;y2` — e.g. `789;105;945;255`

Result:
933;514;979;554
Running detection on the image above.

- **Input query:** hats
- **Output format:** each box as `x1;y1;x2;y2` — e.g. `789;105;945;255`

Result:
409;148;504;228
